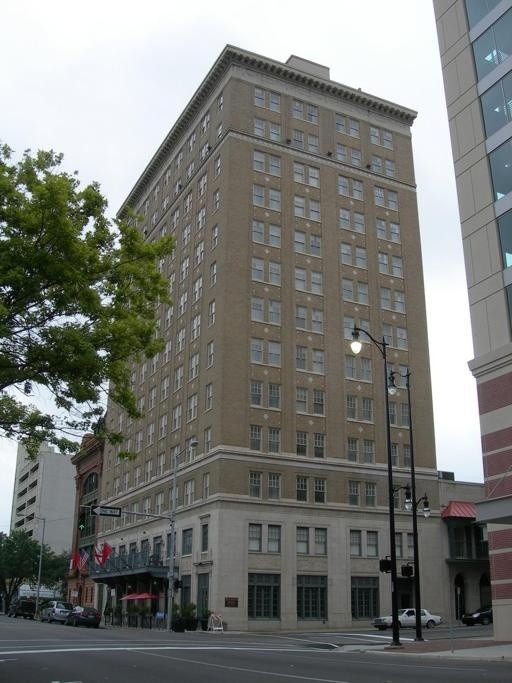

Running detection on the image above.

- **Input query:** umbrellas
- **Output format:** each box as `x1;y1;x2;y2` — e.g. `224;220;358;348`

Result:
128;591;159;599
119;592;139;608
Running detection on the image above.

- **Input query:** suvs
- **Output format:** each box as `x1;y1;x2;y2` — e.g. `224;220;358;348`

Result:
7;596;38;620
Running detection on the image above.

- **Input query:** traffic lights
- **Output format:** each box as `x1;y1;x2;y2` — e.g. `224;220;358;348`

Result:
78;510;85;531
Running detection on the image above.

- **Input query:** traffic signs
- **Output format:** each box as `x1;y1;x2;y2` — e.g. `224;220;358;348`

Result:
90;504;123;518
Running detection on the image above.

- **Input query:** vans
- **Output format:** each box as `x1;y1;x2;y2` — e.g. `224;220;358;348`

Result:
40;600;74;624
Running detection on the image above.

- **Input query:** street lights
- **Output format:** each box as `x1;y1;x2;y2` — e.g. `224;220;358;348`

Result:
164;438;199;631
15;511;46;621
348;323;413;649
384;365;432;642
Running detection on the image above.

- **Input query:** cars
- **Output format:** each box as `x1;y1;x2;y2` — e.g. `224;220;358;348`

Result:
370;607;443;631
64;605;101;629
460;605;493;626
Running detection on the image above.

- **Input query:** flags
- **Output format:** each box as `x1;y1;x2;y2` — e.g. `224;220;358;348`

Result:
71;550;82;569
95;543;109;565
102;541;112;563
80;548;89;568
